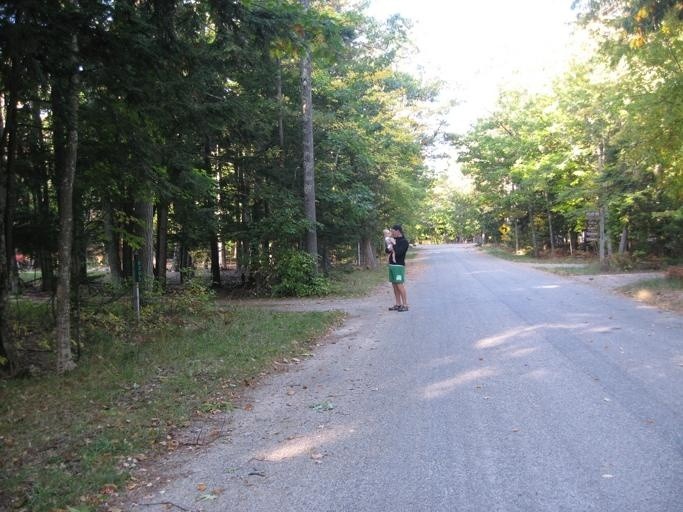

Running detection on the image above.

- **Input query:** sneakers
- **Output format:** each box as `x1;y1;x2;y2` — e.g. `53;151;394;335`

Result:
398;305;407;311
388;304;401;310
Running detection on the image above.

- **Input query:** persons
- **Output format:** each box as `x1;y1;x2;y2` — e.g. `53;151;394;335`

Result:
383;229;397;264
385;224;409;311
